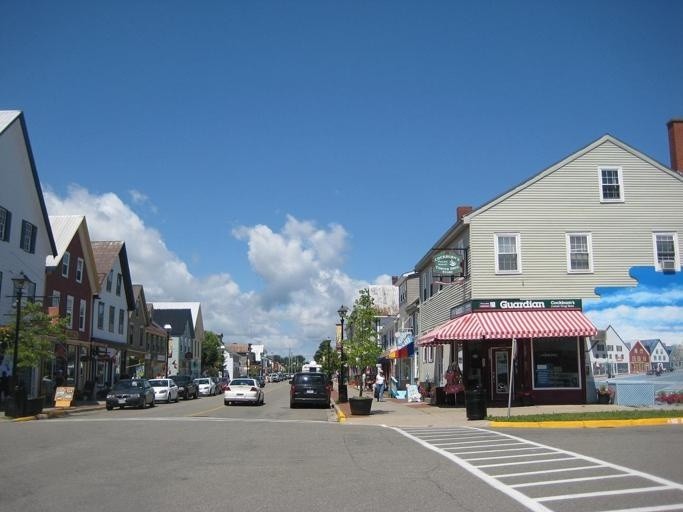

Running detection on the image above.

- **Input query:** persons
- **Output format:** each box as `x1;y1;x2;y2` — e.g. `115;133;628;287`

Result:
156;373;161;379
375;370;387;402
56;369;65;389
361;371;367;391
131;371;138;380
0;371;9;401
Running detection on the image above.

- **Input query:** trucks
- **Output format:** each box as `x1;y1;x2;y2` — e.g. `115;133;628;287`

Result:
301;361;323;374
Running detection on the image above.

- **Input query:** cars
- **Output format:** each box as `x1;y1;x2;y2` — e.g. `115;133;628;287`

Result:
192;377;216;397
212;376;231;395
255;371;294;388
165;375;200;400
105;378;155;411
224;377;265;406
147;377;180;403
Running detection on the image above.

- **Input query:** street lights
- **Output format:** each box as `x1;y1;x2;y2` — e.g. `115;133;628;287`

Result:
5;269;60;418
163;321;172;379
337;305;350;403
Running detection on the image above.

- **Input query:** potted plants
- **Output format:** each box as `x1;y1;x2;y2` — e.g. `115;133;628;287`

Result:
337;289;387;416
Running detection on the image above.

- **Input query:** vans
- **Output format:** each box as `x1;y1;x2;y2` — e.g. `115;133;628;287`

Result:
289;373;333;409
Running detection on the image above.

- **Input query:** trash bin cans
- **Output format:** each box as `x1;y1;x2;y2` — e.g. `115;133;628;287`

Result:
466;390;487;420
